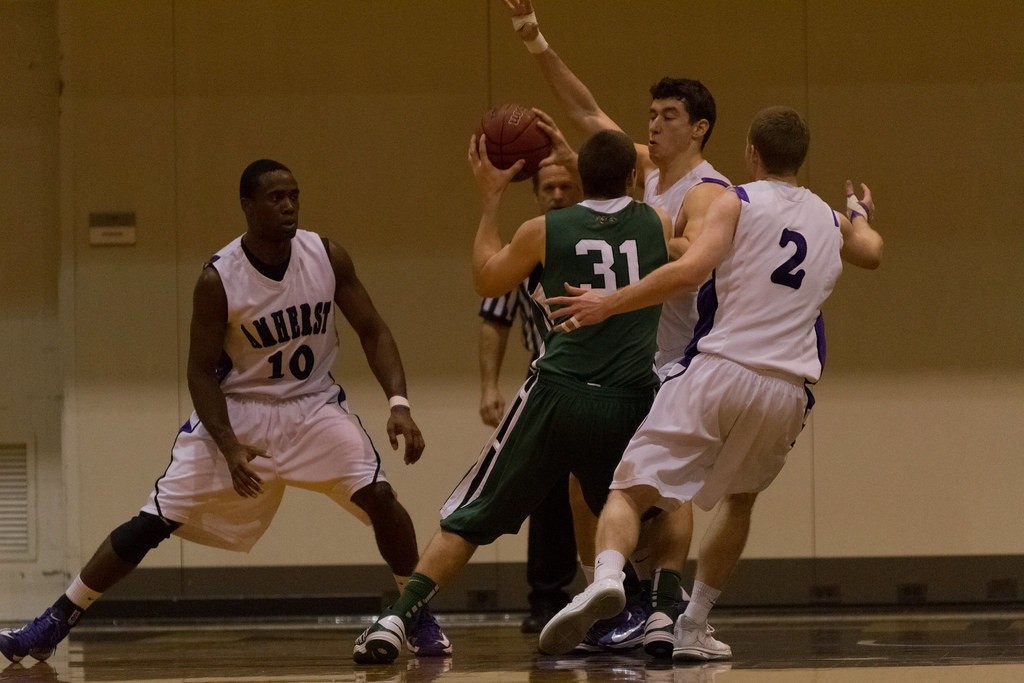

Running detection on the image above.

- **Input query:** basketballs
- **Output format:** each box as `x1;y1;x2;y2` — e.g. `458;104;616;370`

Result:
474;102;554;183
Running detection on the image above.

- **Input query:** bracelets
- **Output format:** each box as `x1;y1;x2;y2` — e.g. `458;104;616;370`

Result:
389;395;411;410
524;31;548;54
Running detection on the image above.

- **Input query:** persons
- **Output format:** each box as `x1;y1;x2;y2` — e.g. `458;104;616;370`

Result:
351;0;884;663
0;157;454;663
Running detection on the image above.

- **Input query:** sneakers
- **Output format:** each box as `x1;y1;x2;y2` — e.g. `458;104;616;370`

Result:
406;606;453;654
0;606;70;663
671;614;731;660
645;612;676;657
572;624;620;651
598;610;645;647
540;571;626;654
352;605;408;665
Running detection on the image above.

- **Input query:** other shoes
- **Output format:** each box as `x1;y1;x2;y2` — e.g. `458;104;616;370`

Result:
523;616;547;631
